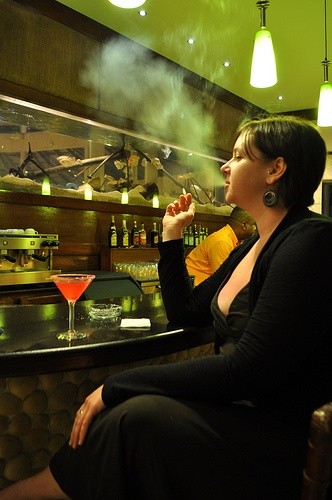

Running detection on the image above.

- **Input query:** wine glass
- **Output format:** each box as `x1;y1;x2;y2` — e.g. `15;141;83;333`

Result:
50;274;96;340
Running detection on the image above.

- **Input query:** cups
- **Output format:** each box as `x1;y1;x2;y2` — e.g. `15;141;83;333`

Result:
0;228;38;234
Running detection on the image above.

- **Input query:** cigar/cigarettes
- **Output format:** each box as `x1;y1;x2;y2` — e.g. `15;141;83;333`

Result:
182;187;188;202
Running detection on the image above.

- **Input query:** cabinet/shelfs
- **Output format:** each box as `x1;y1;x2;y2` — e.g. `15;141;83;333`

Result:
100;246;198;284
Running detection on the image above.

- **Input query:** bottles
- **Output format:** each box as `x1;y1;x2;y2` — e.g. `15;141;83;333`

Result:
151;223;158;247
140;223;146;248
110;215;118;248
132;220;140;247
183;224;209;247
120;219;130;248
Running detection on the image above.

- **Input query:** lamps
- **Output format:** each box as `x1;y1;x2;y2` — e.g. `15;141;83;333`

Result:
317;0;332;127
249;0;278;89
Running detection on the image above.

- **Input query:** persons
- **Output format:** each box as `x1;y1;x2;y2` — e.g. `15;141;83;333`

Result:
185;206;255;287
0;115;332;500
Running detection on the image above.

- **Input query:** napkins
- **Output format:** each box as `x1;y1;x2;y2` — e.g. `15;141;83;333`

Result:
120;318;151;328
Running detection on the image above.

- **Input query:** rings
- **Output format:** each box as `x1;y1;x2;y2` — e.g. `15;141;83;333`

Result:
80;410;83;414
